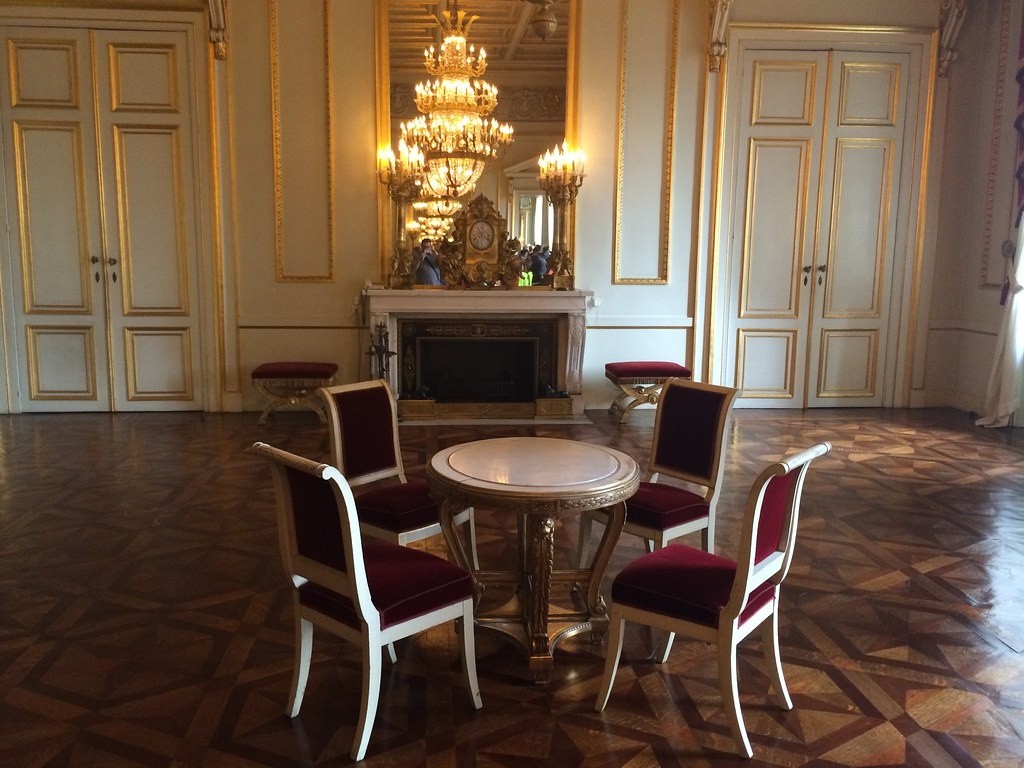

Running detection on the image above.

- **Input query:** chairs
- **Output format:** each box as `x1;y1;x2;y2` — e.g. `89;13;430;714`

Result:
571;378;743;593
318;376;488;590
251;440;484;762
592;439;833;760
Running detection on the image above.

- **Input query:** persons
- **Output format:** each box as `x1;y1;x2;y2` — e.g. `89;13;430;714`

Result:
512;239;551;286
412;238;441;285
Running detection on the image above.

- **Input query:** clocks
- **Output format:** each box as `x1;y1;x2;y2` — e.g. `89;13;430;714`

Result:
455;192;508;281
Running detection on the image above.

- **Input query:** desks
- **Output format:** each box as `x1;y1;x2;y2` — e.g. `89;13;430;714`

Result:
427;436;641;673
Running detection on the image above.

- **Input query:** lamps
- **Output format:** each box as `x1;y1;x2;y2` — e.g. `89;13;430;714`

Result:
538;136;587;290
376;0;512;248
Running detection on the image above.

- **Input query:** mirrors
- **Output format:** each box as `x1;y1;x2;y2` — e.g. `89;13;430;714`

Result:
376;0;586;288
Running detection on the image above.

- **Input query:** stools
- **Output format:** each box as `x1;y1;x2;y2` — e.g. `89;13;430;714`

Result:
603;361;694;425
249;359;337;427
